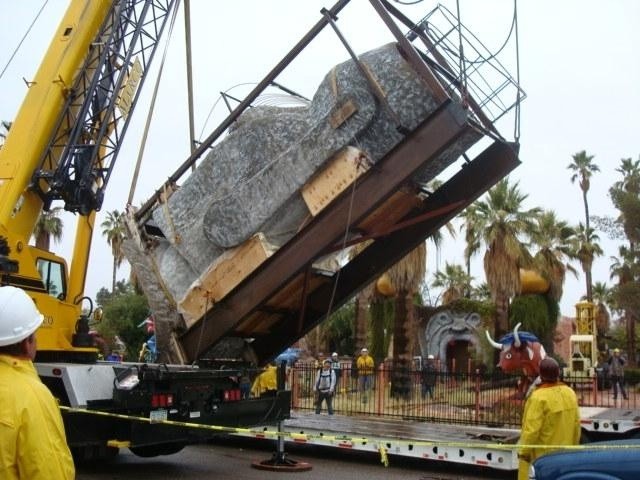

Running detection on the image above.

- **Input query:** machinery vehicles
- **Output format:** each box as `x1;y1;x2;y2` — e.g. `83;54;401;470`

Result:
561;300;611;391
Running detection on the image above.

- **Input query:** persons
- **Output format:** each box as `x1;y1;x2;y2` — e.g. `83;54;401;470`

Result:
312;348;374;415
0;284;76;480
517;358;582;479
420;353;438;399
608;348;628;399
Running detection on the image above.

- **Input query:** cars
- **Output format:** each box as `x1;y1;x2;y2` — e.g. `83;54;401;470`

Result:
529;439;640;480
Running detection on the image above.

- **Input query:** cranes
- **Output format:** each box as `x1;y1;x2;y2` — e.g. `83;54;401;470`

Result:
1;1;292;472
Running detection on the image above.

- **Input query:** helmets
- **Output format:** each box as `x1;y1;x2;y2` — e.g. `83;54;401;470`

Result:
0;285;45;346
539;359;559;380
